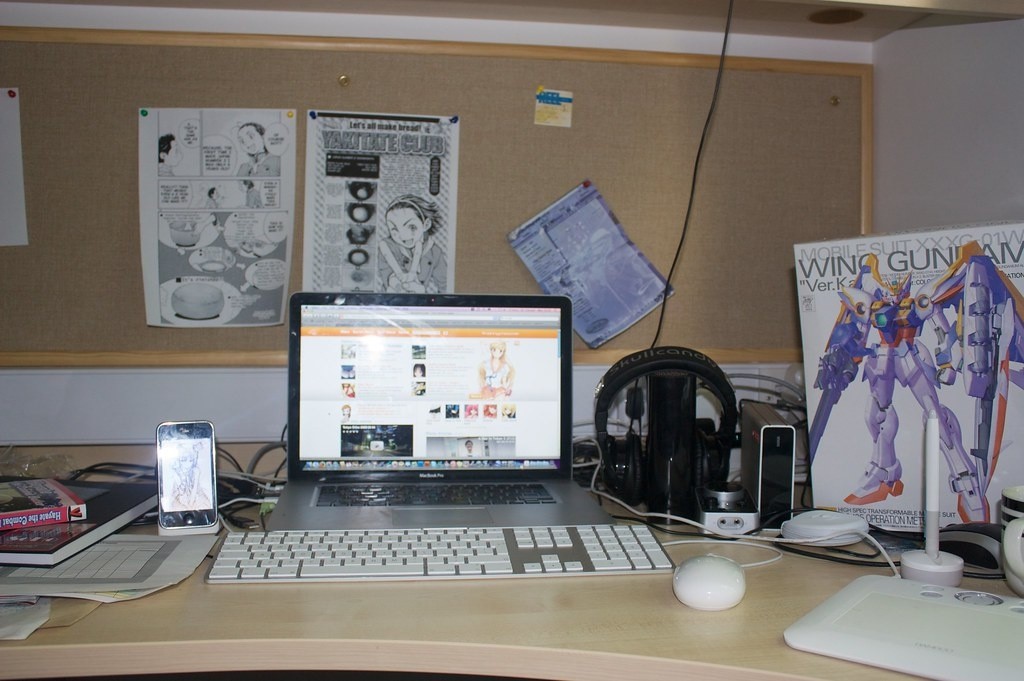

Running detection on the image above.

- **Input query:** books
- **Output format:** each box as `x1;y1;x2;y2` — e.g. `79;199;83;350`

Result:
0;477;87;531
0;594;39;608
0;477;160;567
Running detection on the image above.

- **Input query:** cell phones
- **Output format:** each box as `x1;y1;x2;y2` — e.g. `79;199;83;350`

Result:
155;420;218;529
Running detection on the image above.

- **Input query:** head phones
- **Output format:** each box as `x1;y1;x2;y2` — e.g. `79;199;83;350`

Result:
591;345;738;506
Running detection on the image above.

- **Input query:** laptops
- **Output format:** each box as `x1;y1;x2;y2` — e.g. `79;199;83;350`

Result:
264;291;615;532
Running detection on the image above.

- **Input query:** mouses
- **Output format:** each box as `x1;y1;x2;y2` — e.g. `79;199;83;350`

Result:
937;522;1005;571
672;553;746;612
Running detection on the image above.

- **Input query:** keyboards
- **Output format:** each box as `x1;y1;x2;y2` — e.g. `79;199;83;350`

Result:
204;523;675;585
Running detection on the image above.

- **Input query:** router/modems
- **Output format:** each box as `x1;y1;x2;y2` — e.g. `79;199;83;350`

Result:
740;400;796;531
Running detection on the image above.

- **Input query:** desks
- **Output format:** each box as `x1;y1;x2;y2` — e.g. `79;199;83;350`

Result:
1;470;1023;680
